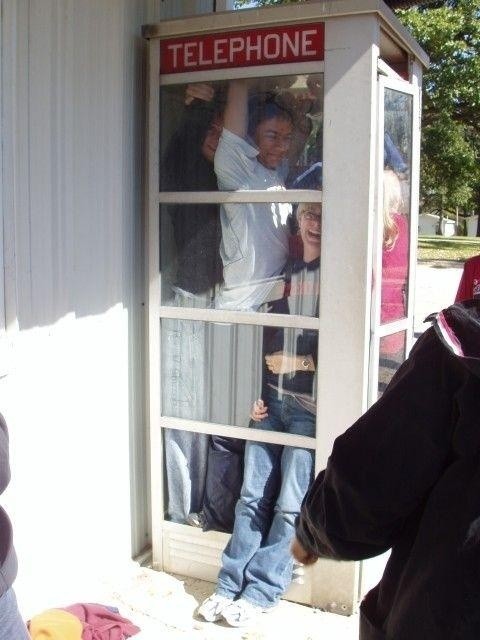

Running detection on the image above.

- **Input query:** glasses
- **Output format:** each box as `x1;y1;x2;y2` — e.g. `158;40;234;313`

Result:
300;209;320;223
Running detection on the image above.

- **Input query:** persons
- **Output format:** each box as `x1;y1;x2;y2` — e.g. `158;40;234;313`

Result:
162;80;411;630
291;255;479;639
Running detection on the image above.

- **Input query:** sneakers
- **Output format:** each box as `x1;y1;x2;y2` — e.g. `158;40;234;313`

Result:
186;509;234;533
219;597;282;628
197;592;237;622
